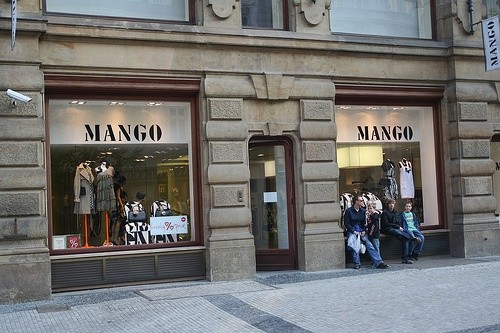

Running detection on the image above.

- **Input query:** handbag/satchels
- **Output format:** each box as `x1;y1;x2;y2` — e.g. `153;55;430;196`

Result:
378;178;391;186
156;202;172;217
348;232;360;253
127;204;146;223
80;186;86;196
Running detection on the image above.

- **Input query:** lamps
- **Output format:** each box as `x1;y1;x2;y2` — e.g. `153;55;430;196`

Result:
265;161;275;177
337;145;383;168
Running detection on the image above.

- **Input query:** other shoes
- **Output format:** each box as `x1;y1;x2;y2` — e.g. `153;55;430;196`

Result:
401;257;413;264
413;237;421;243
377;263;389;269
414;254;419;261
354;265;360;269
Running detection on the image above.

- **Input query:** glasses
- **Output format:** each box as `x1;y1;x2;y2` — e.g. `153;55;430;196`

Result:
358;199;365;203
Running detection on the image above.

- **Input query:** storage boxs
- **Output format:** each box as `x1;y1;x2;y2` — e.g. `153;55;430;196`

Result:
52;234;81;249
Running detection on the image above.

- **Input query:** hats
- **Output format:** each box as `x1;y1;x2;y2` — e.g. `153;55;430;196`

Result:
385;197;396;206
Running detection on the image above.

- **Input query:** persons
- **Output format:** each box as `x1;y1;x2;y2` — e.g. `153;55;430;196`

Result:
73;161;170;246
402;200;425;260
367;203;380;266
382;159;398;200
380;199;421;264
398;158;414;199
344;196;390;269
